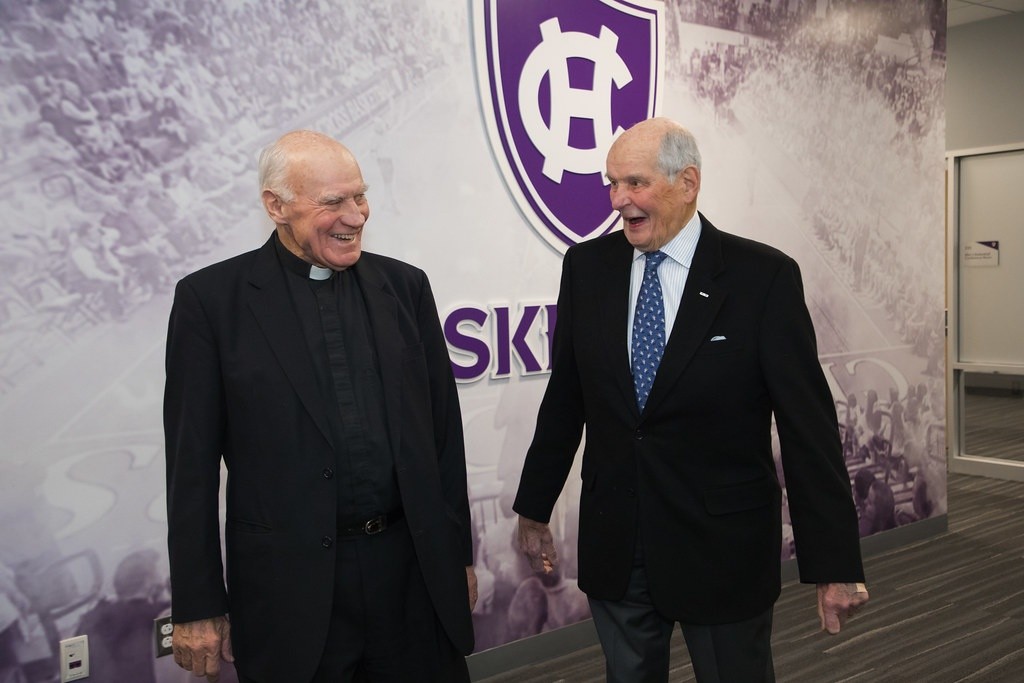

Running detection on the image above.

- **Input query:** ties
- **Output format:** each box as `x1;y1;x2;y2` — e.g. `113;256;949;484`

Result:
631;251;667;414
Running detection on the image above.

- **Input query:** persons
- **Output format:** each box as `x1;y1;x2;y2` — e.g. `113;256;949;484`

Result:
512;116;868;683
163;132;478;683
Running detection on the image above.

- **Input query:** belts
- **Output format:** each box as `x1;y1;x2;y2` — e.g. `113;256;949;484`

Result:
339;507;402;534
